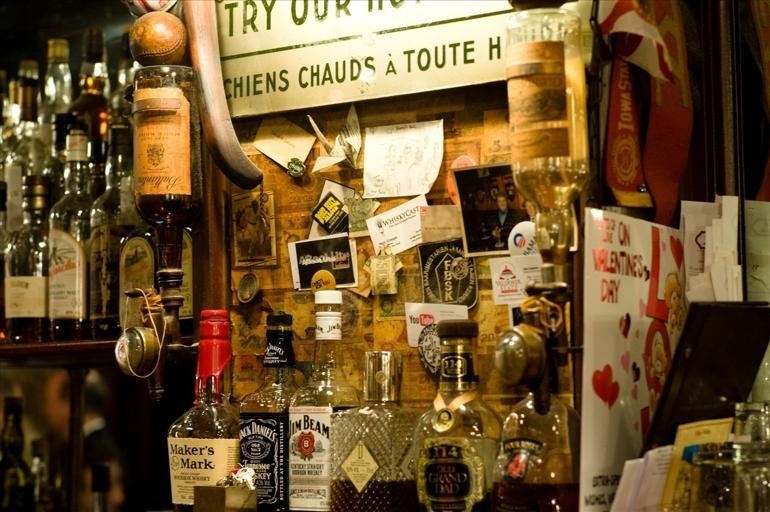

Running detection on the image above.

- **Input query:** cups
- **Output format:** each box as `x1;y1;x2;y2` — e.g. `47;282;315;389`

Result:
675;401;770;512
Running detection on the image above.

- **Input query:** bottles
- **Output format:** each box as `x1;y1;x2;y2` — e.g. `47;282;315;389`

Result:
415;318;499;512
327;347;422;512
1;397;112;512
500;6;585;297
0;23;205;346
165;307;241;512
497;365;583;510
288;284;355;511
240;311;309;512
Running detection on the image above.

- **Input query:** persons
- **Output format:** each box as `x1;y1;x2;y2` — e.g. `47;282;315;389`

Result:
522;200;538;223
484;193;530;251
243;200;271;258
